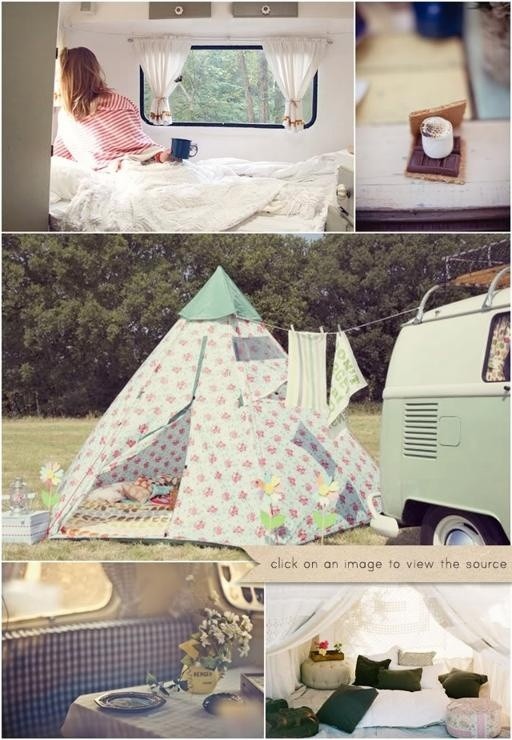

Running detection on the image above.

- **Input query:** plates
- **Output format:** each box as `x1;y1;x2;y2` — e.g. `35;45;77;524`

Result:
94;689;167;715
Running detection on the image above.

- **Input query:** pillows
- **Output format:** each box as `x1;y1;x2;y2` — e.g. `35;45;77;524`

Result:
49;156;97;200
315;646;489;733
89;474;178;505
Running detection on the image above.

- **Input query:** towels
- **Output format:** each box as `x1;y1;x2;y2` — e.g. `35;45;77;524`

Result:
287;330;327;413
323;333;368;428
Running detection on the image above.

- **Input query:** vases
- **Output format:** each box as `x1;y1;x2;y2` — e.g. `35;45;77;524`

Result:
180;664;218;695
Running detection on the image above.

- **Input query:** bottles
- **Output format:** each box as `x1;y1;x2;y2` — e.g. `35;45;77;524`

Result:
7;476;29;516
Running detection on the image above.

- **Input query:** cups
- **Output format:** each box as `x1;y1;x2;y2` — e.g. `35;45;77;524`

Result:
169;137;200;160
419;116;455;160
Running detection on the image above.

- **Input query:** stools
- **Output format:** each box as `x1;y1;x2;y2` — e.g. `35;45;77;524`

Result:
301;659;354;690
446;698;502;738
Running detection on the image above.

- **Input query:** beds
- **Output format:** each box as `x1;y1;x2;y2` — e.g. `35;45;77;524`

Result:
48;144;355;232
266;631;511;739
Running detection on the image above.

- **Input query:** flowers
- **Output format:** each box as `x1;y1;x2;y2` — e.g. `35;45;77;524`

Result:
319;640;329;649
40;462;65;511
258;474;286;546
179;590;254;673
313;482;339;546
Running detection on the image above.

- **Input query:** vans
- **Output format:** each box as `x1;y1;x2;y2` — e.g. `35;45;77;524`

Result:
376;274;511;555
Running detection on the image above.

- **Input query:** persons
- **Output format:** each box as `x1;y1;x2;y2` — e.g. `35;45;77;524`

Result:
51;47;186;170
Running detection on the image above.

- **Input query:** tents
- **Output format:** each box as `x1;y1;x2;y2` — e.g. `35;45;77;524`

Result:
47;266;383;547
